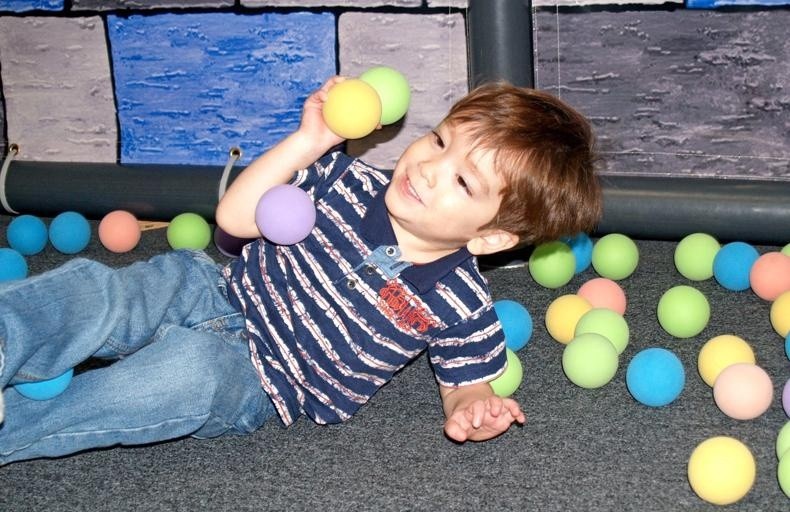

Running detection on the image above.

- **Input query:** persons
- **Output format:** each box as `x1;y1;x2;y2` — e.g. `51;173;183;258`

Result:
0;75;604;469
